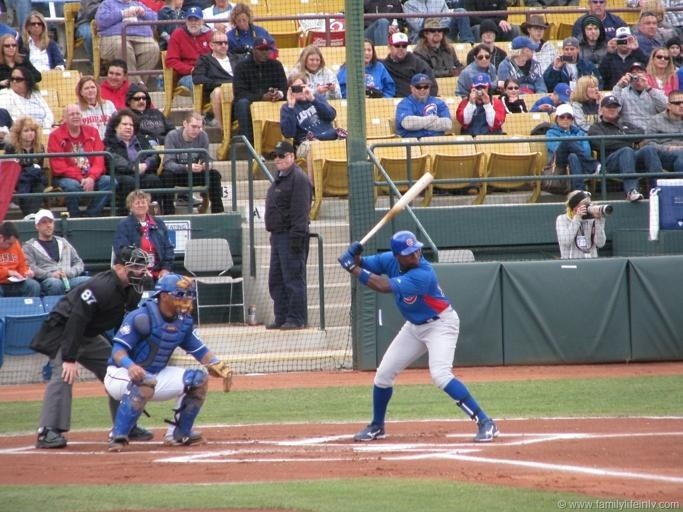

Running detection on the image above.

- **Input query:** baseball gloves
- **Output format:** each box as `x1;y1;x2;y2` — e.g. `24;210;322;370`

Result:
207;359;234;393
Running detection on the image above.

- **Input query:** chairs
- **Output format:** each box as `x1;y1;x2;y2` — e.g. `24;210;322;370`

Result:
1;0;248;356
224;1;681;218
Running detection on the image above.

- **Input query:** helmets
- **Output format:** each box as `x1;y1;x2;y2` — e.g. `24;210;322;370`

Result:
390;230;425;257
114;244;149;266
153;274;196;300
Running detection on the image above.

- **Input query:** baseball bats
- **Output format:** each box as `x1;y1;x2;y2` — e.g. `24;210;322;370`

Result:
359;171;434;247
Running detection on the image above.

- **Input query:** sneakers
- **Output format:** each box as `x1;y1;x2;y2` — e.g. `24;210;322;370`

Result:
473;416;500;443
36;427;68;449
163;431;207;448
280;322;305;330
123;423;154;442
625;189;644;203
592;164;602;175
264;322;283;330
353;423;387;443
106;433;129;452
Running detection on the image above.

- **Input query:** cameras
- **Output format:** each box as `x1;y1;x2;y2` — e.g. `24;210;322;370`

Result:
617;40;627;45
560;56;573;61
477;90;482;96
291;86;302;92
632;75;639;81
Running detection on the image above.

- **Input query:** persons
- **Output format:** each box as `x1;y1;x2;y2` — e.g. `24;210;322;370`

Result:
337;231;501;443
30;244;153;448
103;272;231;451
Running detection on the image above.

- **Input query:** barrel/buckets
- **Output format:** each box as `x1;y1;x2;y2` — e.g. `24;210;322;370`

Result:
650;177;683;229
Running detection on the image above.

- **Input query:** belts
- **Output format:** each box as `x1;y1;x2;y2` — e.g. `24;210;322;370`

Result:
411;316;441;325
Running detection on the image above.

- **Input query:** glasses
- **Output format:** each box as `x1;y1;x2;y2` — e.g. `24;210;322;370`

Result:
130;96;147;102
476;54;491;61
668;101;683;105
2;44;17;48
9;77;26;83
28;22;43;26
424;28;444;33
505;86;520;90
271;154;289;160
391;43;409;48
654;55;669;60
591;1;605;4
558;116;571;120
411;84;431;90
211;41;228;45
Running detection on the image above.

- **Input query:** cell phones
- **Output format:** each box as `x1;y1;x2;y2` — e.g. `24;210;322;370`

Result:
326;83;332;86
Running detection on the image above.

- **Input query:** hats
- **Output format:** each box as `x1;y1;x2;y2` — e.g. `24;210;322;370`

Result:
184;7;204;21
479;18;499;39
471;73;489;88
388;32;409;45
563;36;579;50
410;73;433;85
615;26;632;39
521;15;550;36
418;16;449;39
34;208;54;224
666;37;682;50
553;82;572;104
511;35;540;52
253;37;273;52
629;61;647;75
270;141;294;155
601;95;621;109
554;103;574;119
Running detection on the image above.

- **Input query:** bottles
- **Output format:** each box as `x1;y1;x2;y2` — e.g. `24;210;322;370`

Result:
60;271;69;292
151;201;160;215
247;304;256;324
156;74;164;92
391;18;398;30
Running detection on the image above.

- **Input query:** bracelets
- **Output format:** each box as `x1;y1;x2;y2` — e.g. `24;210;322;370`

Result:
121;356;133;368
209;358;218;363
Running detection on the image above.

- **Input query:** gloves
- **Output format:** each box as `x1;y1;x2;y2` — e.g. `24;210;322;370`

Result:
337;250;358;273
346;240;364;257
